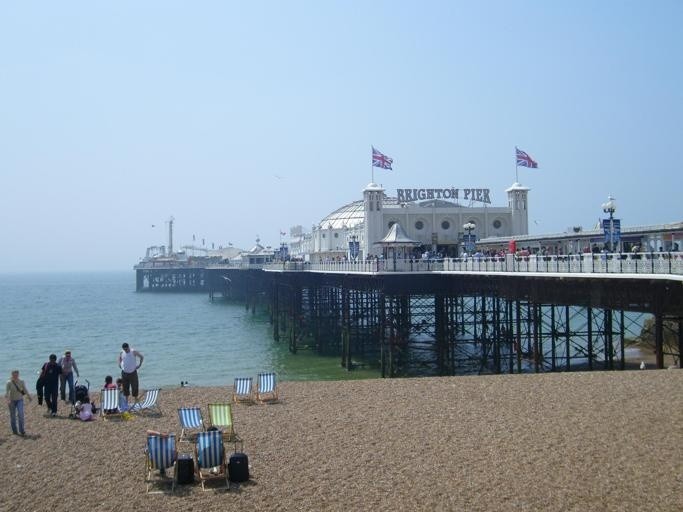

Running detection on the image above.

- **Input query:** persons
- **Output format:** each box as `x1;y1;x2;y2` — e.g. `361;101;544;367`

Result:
409;249;445;271
461;243;681;262
281;253;387;269
5;370;33;435
36;351;94;420
103;342;143;403
196;426;226;473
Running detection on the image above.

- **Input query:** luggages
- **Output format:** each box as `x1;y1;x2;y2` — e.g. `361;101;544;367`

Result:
228;441;249;483
178;455;194;484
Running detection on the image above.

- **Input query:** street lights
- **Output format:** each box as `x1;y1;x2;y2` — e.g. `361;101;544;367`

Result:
463;223;475;255
601;194;618;253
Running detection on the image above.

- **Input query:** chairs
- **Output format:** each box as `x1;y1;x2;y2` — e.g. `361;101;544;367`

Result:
194;430;231;492
257;372;278;404
144;433;179;495
177;407;206;443
99;388;124;422
128;388;162;418
207;403;242;443
231;378;253;406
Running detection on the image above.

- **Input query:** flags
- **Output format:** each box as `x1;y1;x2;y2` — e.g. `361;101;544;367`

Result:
372;144;393;170
516;147;537;169
